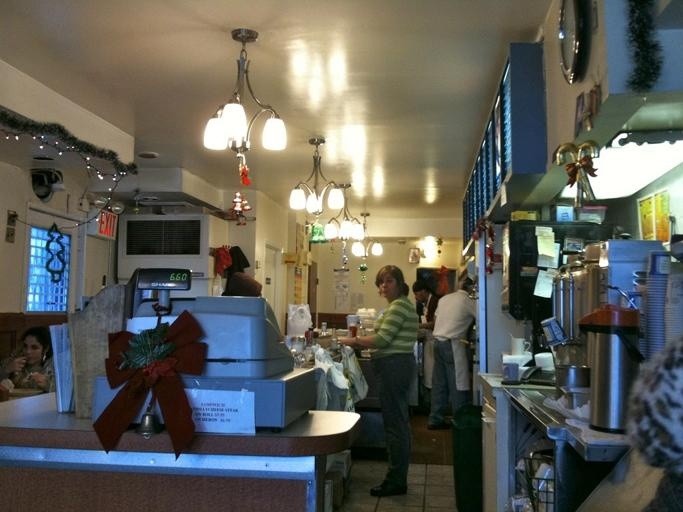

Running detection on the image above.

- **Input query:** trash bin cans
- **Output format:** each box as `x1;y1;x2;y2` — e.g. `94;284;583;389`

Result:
451;404;483;512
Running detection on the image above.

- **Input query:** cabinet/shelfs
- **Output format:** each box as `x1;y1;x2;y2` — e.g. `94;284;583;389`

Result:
118;214;209;279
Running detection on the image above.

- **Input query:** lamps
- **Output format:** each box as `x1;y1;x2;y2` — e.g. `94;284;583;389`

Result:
288;137;384;286
202;28;287;187
89;192;124;215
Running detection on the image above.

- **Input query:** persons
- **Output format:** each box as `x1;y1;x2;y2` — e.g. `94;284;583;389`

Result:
0;325;58;400
337;264;422;498
409;280;438;408
426;279;478;432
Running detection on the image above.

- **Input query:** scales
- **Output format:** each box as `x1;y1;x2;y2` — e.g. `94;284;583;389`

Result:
125;268;294;379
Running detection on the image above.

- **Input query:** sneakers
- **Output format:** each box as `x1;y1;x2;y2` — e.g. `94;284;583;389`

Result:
369;481;408;497
427;418;453;431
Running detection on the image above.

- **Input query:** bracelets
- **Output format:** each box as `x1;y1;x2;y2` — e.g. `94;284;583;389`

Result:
355;336;361;345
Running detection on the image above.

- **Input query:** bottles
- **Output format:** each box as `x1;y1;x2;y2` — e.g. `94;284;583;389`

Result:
298;322;338;351
502;362;519;383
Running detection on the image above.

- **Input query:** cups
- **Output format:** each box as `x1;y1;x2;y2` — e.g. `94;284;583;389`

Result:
541;317;569;346
640;252;683;360
510;337;532;356
